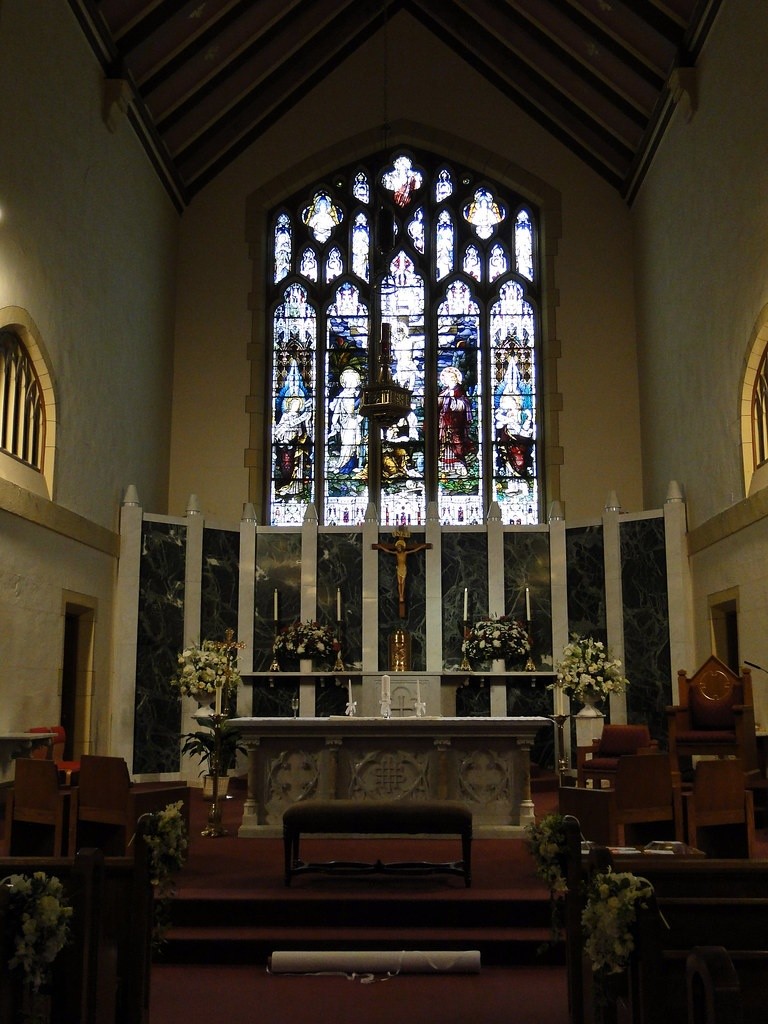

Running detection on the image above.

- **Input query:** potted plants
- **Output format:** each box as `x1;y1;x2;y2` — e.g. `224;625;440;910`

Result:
179;719;249;800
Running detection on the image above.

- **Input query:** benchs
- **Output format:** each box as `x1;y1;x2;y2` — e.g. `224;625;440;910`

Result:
280;797;475;886
0;810;166;1024
543;820;768;1023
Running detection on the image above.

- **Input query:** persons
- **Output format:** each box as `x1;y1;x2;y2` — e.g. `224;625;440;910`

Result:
376;544;428;602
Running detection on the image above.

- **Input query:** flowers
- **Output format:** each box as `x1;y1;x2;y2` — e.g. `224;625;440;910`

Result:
459;614;538;670
0;870;75;993
553;631;630;704
169;640;241;698
522;812;563;955
274;621;338;654
579;863;652;977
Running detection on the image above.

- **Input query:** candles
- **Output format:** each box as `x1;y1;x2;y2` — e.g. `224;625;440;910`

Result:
464;588;468;621
348;678;352;703
416;679;421;705
382;674;391;715
274;589;278;620
526;588;531;619
337;589;342;620
556;674;564;717
214;676;223;716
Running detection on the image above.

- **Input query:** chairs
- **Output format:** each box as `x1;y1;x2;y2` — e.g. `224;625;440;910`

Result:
4;754;191;868
560;654;757;857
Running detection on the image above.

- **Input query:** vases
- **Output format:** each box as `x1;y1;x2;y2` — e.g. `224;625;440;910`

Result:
573;688;609;716
189;685;219;717
